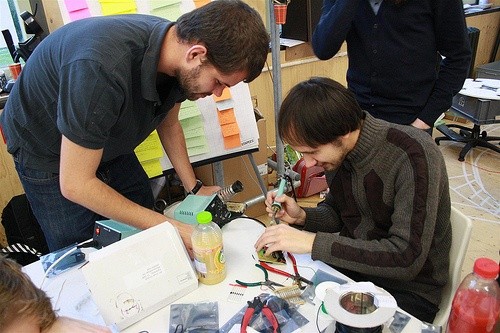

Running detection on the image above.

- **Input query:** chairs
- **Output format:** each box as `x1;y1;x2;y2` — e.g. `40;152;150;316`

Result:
435;27;500;162
434;206;472;333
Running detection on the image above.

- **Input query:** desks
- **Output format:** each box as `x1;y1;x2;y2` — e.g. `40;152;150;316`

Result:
21;215;438;333
465;4;500;69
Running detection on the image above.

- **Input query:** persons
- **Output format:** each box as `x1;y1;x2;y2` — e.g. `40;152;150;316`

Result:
254;77;452;323
0;0;270;260
0;255;111;333
311;0;473;135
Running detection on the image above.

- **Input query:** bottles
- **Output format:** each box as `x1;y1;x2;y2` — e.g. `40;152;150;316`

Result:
190;211;227;285
444;258;500;333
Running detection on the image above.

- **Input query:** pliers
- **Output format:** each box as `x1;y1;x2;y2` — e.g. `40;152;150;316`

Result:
241;296;281;333
260;252;313;290
236;264;285;290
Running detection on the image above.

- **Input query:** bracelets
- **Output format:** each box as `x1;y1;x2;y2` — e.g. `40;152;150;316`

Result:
186;179;202;196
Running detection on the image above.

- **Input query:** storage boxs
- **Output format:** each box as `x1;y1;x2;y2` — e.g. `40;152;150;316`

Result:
195;109;269;217
451;93;500;125
